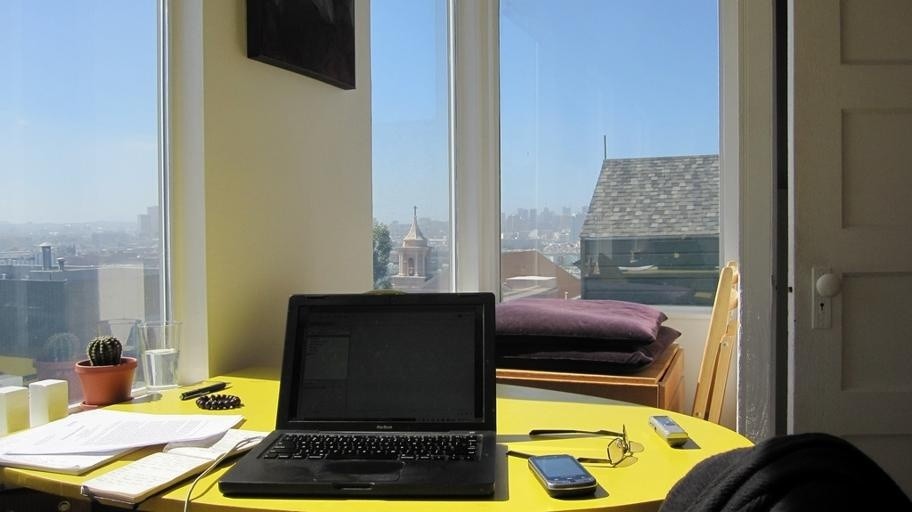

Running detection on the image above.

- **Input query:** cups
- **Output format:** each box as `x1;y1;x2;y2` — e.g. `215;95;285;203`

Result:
136;321;181;390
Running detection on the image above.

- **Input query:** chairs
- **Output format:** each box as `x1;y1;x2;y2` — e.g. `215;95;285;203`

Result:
687;261;742;417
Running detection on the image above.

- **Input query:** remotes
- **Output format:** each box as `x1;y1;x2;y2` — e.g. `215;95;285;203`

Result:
648;415;691;449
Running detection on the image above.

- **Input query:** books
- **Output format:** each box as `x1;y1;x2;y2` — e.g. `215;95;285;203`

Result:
0;410;270;504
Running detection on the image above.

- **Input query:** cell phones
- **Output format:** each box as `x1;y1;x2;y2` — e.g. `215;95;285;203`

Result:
528;453;598;498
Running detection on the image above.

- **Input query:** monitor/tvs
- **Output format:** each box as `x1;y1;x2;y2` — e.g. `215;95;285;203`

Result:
246;0;355;91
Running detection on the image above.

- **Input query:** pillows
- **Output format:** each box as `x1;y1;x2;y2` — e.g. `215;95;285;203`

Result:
493;298;682;368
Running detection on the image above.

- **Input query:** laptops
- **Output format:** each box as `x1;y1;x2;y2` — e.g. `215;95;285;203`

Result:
217;292;496;499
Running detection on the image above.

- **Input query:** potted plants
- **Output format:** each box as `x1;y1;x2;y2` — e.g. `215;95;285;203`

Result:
33;332;84;404
75;338;139;402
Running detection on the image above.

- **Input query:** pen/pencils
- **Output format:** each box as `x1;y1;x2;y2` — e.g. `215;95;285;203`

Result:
180;383;226;400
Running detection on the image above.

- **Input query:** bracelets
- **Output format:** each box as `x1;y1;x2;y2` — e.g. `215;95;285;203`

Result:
196;394;240;410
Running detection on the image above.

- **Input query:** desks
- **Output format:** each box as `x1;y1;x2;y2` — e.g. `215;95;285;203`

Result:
493;345;684;412
4;374;756;512
